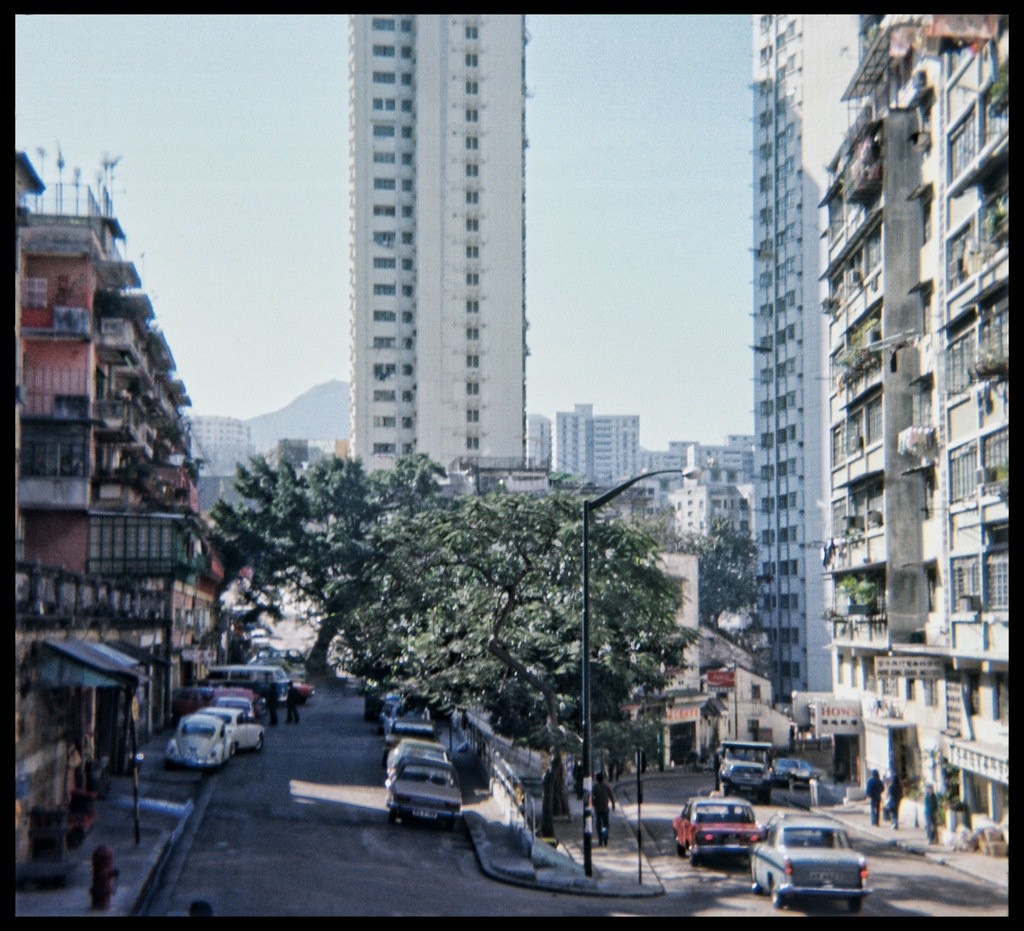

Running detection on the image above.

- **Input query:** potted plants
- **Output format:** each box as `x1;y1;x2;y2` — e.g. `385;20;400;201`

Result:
840;575;875;614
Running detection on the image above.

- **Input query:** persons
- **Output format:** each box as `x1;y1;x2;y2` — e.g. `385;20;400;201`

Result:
714;753;721;790
884;775;903;830
189;901;212;916
656;748;664;772
264;680;278;727
689;750;700;772
594;772;614;846
867;770;886;826
283;679;300;724
608;754;624;782
925;784;936;843
788;776;795;794
641;751;647;773
809;773;819;806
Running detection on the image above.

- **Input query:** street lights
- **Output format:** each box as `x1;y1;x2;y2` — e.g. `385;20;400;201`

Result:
583;466;702;875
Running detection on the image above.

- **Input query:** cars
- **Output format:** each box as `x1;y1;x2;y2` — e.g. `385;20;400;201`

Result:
718;740;775;805
772;759;821;788
386;739;449;777
746;812;875;912
165;713;232;774
672;796;763;868
213;696;255;721
196;706;264;752
170;665;316;723
385;755;463;830
229;606;308;681
383;718;443;768
364;684;431;735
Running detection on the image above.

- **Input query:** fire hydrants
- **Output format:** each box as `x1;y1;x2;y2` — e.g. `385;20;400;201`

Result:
90;845;119;910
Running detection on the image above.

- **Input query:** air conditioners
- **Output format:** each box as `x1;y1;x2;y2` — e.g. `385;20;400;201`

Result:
849;435;860;453
975;467;990;488
848;268;859;284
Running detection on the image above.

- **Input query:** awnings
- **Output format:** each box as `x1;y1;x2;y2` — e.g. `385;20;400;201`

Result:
20;639;170;691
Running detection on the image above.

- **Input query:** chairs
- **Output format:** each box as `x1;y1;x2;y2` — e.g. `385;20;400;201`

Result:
697;813;722;823
724;813;745;821
432;776;447;787
787;836;826;846
